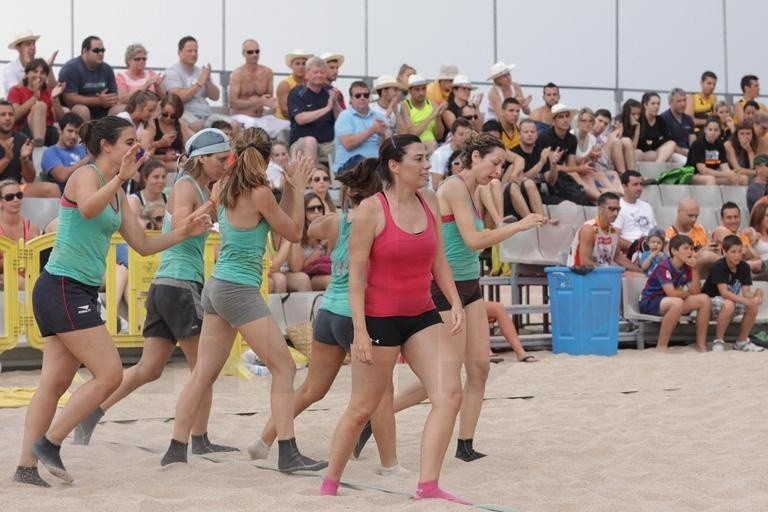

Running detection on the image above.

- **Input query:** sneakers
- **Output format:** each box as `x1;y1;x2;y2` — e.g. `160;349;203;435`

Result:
732;337;764;352
489;353;505;363
712;338;725;352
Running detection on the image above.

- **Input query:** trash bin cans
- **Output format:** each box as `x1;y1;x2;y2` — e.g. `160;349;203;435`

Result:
544;266;625;356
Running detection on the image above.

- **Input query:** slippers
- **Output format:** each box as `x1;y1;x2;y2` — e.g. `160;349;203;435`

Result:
518;354;540;363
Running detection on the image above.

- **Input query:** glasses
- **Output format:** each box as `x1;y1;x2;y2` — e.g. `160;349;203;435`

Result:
90;47;106;53
134;57;147;61
314;176;329;182
602;205;622;211
162;113;177;119
1;191;24;202
306;204;325;212
246;49;260;54
461;114;479;121
451;160;462;167
355;92;369;99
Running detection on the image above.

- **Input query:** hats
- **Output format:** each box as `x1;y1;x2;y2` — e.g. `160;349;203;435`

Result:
647;225;666;240
485;60;515;82
284;48;315;69
320;52;345;68
407;74;430;89
752;110;768;129
448;75;480;91
433;64;460;81
544;103;579;124
753;154;768;165
370;74;401;93
7;30;40;50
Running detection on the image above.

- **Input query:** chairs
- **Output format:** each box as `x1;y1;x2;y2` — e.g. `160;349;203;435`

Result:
18;197;61;234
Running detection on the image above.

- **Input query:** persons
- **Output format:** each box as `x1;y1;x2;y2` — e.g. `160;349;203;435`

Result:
1;32;388;295
9;115;214;489
246;155;415;477
74;128;239;455
372;60;768;361
349;128;548;460
317;134;474;504
154;126;330;473
